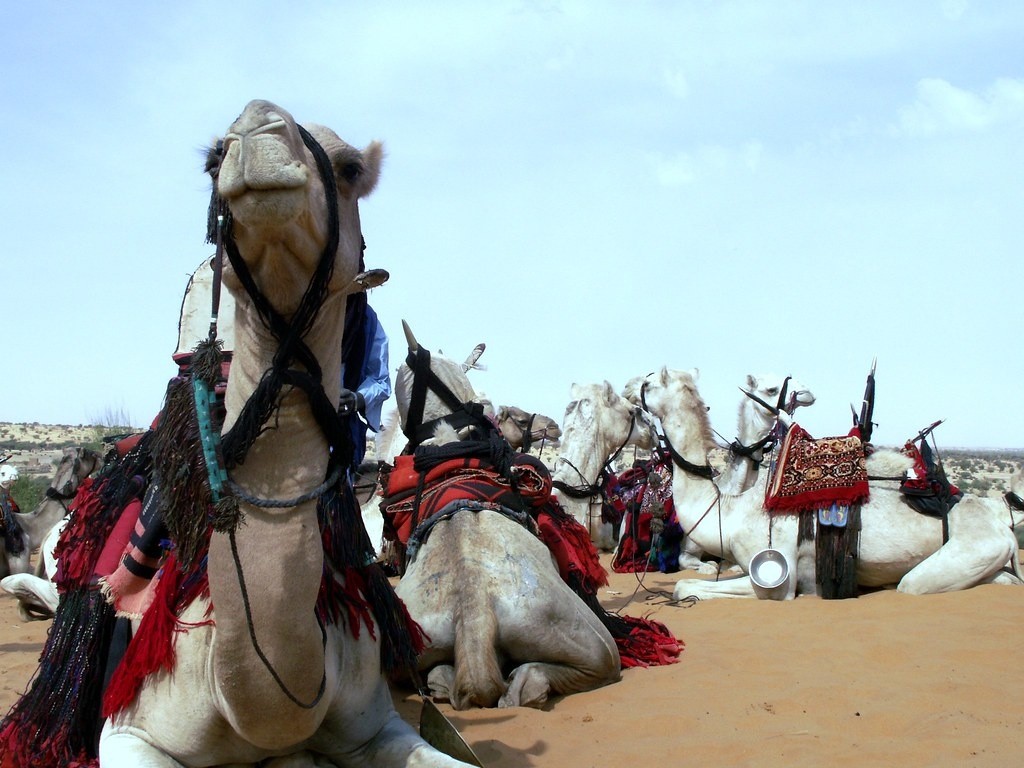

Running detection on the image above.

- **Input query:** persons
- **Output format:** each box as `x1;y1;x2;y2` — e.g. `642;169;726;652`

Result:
338;303;389;464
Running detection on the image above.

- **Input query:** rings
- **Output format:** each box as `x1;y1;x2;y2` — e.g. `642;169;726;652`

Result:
344;404;347;411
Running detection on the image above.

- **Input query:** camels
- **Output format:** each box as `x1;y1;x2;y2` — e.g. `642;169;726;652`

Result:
394;344;622;714
1;98;477;768
486;364;1024;601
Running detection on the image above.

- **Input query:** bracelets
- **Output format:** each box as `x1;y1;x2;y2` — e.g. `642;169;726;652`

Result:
350;392;357;412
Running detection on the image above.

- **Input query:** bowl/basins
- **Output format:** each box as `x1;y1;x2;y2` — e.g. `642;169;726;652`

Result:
749;549;789;588
